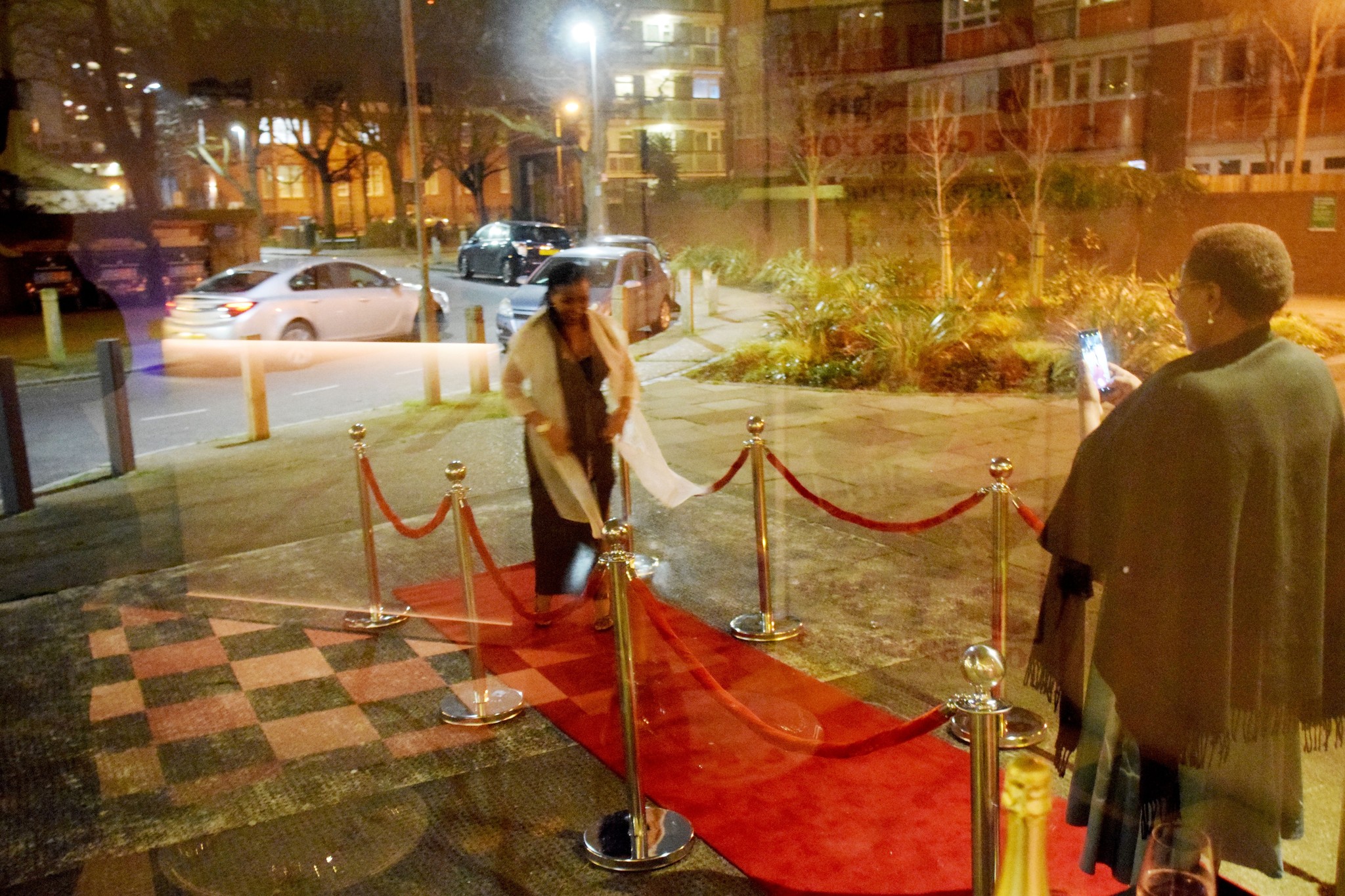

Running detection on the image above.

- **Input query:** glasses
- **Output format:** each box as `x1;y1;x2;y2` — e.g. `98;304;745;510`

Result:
1168;279;1212;305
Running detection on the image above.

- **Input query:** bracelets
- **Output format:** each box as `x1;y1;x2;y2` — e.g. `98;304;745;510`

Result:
616;407;629;414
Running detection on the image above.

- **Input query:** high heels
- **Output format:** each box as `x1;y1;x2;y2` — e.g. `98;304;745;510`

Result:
593;594;614;629
535;591;555;625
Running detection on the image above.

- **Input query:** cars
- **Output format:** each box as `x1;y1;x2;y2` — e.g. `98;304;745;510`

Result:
162;255;452;359
572;235;682;295
492;246;671;356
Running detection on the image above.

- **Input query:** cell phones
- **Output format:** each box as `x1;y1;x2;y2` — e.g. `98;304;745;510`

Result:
1078;326;1115;395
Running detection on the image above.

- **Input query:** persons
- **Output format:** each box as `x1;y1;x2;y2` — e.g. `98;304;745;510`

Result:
1037;222;1345;886
0;65;132;893
504;262;633;631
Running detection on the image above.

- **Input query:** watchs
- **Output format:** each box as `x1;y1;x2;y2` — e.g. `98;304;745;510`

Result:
536;420;552;436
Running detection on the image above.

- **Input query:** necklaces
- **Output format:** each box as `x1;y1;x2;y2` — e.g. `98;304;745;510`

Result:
563;319;580;325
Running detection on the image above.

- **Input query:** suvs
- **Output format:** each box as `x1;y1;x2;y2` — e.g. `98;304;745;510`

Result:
458;218;568;288
